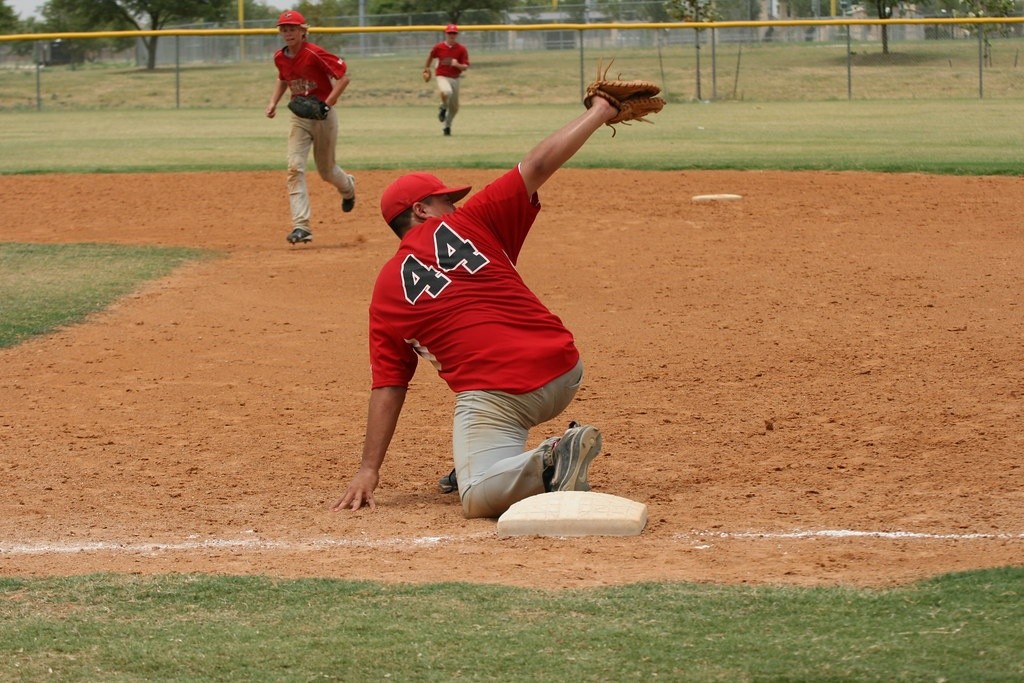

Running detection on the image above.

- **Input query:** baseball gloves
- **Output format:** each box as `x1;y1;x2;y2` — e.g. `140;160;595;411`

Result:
286;94;329;121
422;68;431;83
582;58;669;137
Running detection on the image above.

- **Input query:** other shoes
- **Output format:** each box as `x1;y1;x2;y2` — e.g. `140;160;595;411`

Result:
341;176;355;212
443;127;451;136
287;229;312;243
439;109;446;121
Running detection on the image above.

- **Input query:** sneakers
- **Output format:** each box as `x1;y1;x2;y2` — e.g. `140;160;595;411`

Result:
550;421;602;491
438;467;457;494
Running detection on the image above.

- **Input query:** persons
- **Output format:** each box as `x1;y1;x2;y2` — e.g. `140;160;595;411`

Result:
329;96;616;518
424;27;468;132
267;11;355;245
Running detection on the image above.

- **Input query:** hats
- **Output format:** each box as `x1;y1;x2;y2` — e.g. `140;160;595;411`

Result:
277;11;305;26
380;173;472;225
446;24;458;33
